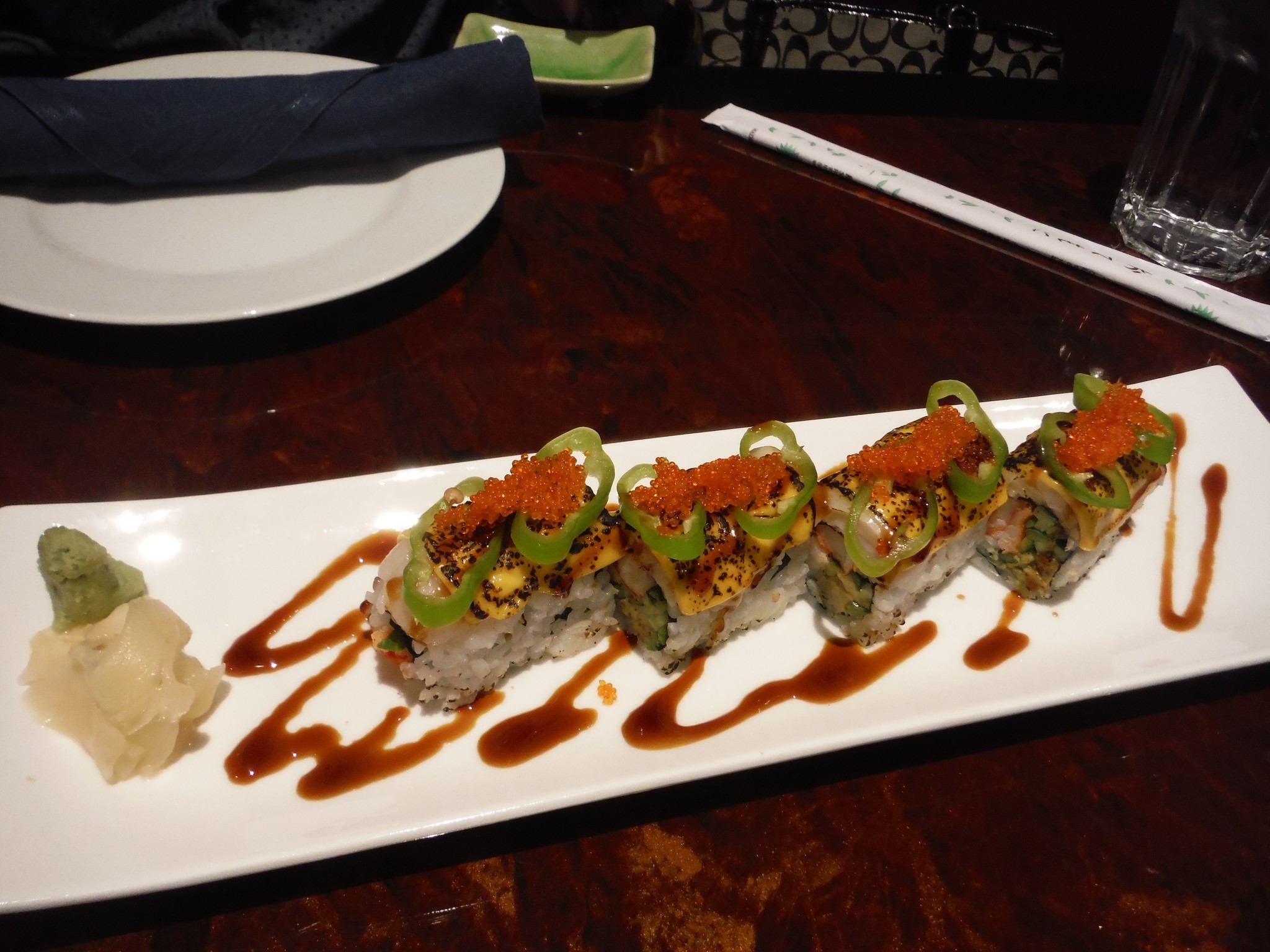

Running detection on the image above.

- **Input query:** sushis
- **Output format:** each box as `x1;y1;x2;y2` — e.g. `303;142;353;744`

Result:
367;379;1177;709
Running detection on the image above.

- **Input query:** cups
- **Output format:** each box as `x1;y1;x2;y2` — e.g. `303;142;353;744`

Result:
1111;5;1268;281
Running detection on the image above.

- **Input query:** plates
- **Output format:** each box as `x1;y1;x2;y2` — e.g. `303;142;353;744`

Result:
3;42;506;330
3;361;1270;913
451;11;657;90
